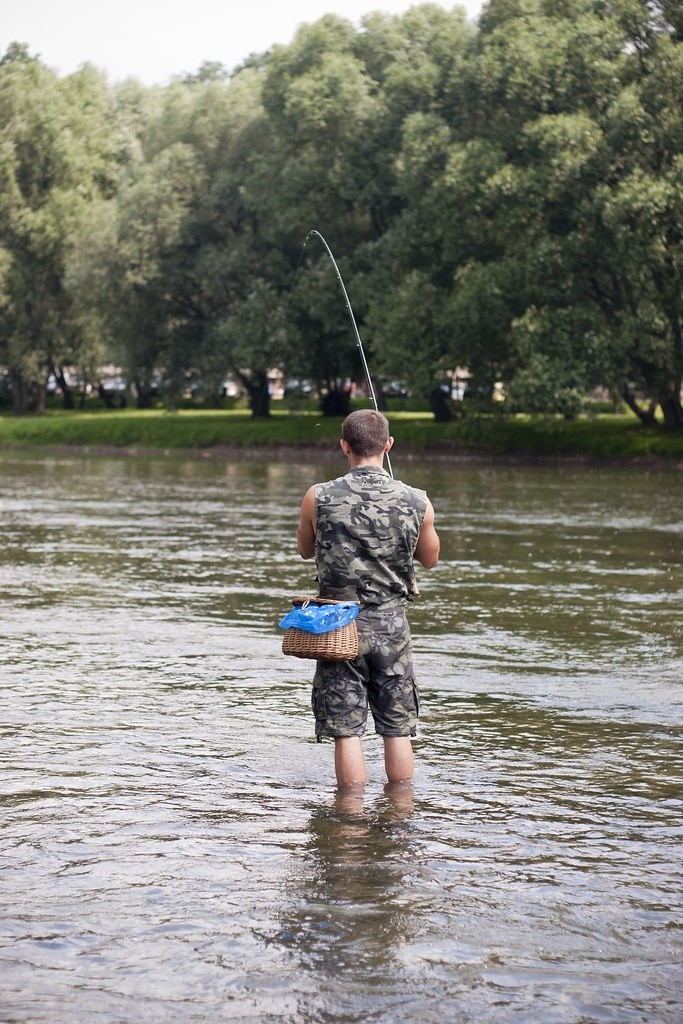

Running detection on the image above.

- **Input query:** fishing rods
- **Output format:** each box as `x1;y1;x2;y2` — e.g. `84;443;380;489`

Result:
302;230;421;600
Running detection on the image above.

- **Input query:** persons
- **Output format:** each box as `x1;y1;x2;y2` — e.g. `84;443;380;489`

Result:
296;410;440;785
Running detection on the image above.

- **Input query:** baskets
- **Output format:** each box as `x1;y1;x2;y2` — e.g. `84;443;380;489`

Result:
282;597;360;661
